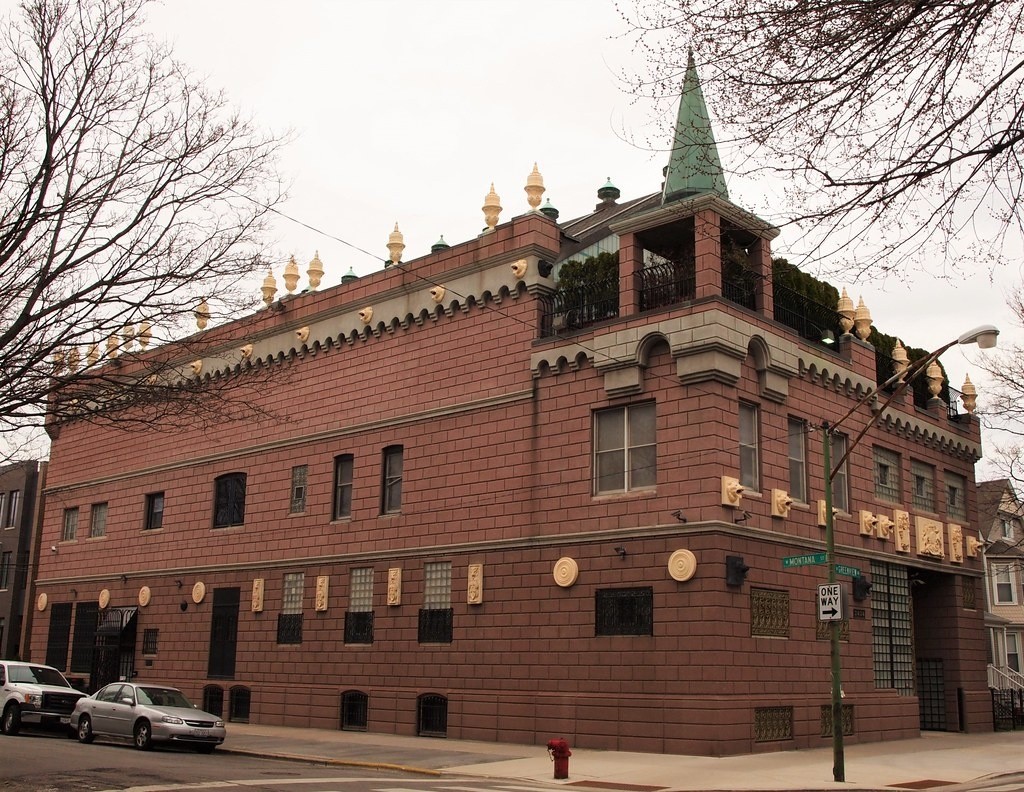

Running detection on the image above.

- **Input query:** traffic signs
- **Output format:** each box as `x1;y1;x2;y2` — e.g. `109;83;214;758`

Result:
818;582;844;622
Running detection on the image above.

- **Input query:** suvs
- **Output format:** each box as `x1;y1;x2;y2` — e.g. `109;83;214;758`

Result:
0;660;90;736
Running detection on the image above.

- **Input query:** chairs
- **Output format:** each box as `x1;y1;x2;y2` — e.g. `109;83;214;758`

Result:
153;696;163;705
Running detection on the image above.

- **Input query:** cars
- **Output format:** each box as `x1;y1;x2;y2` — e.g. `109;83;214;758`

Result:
69;682;226;755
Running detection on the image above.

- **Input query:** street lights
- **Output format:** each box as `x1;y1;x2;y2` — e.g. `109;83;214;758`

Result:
819;322;1001;781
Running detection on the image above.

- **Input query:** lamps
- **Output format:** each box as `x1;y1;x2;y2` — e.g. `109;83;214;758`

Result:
820;329;836;346
